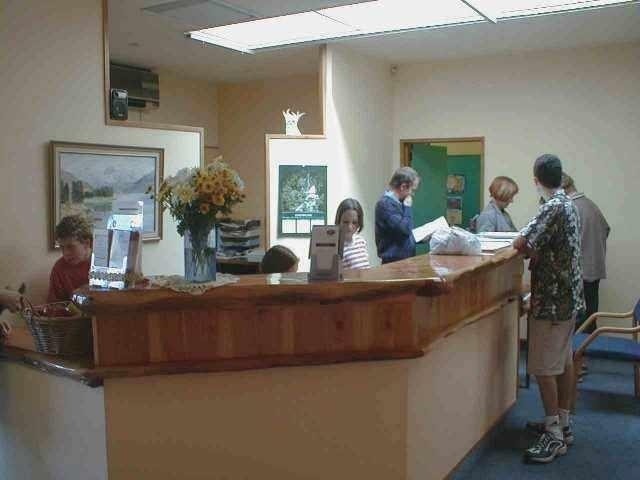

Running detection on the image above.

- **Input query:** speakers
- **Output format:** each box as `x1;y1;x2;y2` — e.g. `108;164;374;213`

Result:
110;88;129;120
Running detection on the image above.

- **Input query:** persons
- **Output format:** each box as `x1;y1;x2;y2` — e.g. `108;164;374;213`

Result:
334;198;371;271
374;167;432;266
256;245;298;274
48;214;94;305
478;176;527;258
512;153;582;463
559;172;611;383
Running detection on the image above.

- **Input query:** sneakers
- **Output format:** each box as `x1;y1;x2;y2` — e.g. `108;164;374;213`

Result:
525;431;567;463
526;421;574;444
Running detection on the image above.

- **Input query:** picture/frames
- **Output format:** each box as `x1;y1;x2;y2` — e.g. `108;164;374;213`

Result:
277;164;327;238
49;140;164;250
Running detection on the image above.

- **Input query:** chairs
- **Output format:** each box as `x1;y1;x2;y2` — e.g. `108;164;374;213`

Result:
570;299;639;415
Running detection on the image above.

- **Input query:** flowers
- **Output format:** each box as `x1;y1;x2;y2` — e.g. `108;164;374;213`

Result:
145;155;246;275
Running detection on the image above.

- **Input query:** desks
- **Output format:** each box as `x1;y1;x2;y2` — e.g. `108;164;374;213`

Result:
215;251;265;273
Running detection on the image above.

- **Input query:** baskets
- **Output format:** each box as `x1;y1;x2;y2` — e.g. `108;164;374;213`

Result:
20;295;91;355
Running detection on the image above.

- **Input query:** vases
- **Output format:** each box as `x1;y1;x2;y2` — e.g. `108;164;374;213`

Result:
182;225;216;282
